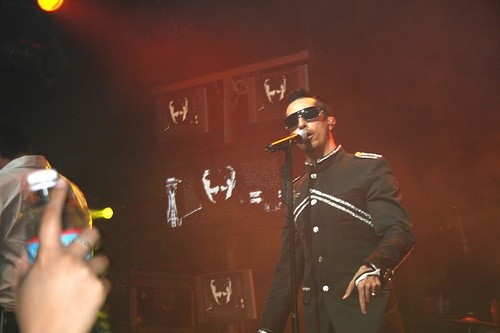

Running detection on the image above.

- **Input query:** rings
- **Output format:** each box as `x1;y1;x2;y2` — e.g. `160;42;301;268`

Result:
370;292;377;298
75;237;91;250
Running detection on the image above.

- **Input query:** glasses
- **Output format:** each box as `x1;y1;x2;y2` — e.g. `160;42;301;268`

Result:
283;106;324;130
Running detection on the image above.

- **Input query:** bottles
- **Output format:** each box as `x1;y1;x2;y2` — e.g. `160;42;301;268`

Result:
25;168;95;264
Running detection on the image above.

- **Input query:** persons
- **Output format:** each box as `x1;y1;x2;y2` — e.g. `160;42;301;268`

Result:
12;177;111;333
255;88;415;333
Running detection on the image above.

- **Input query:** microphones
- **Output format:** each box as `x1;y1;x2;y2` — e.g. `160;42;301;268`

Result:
264;130;307;154
